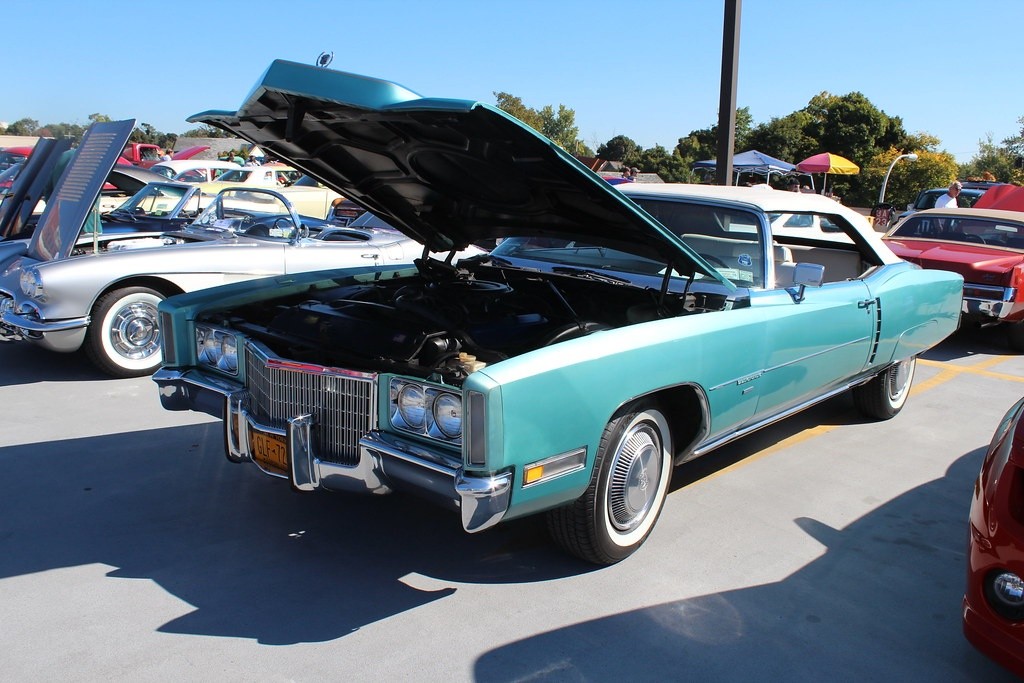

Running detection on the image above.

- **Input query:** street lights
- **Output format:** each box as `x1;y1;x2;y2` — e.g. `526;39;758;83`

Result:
879;154;918;204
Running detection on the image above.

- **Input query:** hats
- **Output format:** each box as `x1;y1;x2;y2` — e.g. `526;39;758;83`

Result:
630;167;641;173
787;177;800;185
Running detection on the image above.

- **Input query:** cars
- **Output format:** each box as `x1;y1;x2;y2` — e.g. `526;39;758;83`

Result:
0;51;965;570
881;180;1024;338
961;397;1024;682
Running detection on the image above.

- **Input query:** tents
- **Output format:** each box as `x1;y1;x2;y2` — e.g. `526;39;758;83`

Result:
690;150;814;193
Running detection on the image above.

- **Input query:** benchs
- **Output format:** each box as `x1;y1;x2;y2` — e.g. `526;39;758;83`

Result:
682;233;862;287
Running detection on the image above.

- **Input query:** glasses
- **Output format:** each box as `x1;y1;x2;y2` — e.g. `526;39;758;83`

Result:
955;188;962;192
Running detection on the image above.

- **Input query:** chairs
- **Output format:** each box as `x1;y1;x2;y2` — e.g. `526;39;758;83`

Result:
709;243;799;289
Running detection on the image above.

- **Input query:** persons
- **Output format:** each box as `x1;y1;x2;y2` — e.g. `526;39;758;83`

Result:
160;148;174;179
224;152;285;183
622;167;640;182
935;180;961;230
746;176;810;194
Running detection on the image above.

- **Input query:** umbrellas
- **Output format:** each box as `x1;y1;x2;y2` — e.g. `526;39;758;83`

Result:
796;152;860;195
